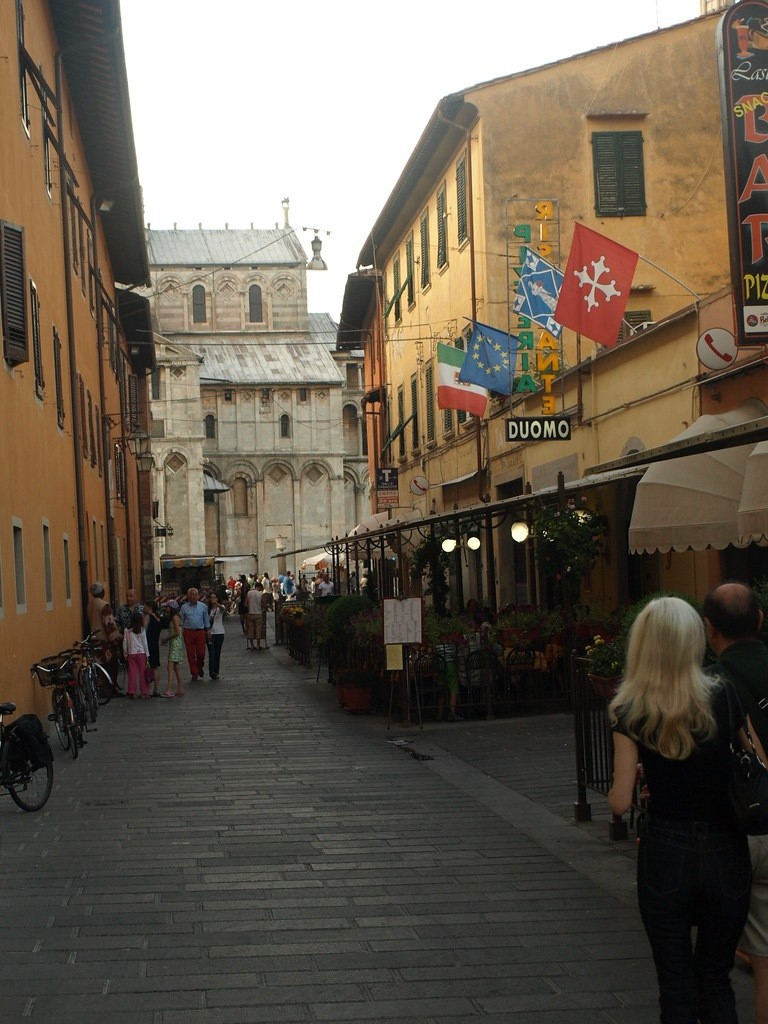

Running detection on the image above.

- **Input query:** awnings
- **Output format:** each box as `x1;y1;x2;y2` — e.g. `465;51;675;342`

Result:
348;507;426;560
629;402;768;556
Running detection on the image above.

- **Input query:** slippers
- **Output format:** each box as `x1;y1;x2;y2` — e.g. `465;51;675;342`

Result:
250;646;266;650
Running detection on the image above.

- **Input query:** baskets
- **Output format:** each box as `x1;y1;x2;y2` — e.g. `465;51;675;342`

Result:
34;656;76;687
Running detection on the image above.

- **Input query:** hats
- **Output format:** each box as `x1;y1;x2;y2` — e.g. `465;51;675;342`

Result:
161;599;178;608
234;581;242;590
90;583;104;595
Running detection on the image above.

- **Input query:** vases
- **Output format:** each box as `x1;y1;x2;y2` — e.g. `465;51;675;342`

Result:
587;673;623;700
347;686;375;711
294;616;303;625
334;682;360;707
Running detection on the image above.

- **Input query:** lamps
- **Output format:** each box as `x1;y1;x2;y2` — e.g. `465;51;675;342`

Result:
510;520;539;545
442;536;461;552
463;536;482;551
302;235;329;270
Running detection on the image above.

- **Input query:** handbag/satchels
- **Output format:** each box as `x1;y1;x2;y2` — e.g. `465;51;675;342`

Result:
236;596;241;604
145;660;154;684
209;606;217;629
717;678;768;836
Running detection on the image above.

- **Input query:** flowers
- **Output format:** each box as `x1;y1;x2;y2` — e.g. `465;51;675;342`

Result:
578;633;625;677
288;603;305;617
524;495;610;579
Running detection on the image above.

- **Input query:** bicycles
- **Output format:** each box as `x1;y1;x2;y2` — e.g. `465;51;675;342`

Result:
30;630;116;759
0;701;54;811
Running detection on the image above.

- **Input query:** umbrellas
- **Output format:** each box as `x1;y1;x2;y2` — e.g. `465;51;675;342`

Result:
303;548;361;565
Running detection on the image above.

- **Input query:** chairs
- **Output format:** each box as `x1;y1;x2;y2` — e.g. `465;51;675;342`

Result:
503;645;538;717
413;651;450;722
454;643;495;718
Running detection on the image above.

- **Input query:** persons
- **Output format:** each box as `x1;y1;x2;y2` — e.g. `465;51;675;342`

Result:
88;581;227;697
704;582;767;1024
227;570;367;654
608;594;767;1024
435;598;627;723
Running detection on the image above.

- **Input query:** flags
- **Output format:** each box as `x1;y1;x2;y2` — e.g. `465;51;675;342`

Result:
513;250;566;340
458;324;518;396
436;343;491;420
553;222;639;349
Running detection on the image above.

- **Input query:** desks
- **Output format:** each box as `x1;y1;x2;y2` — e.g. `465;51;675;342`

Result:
467;646;548;717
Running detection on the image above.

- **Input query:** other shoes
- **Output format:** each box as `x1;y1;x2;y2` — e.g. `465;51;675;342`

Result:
447;712;464;722
191;669;204;681
